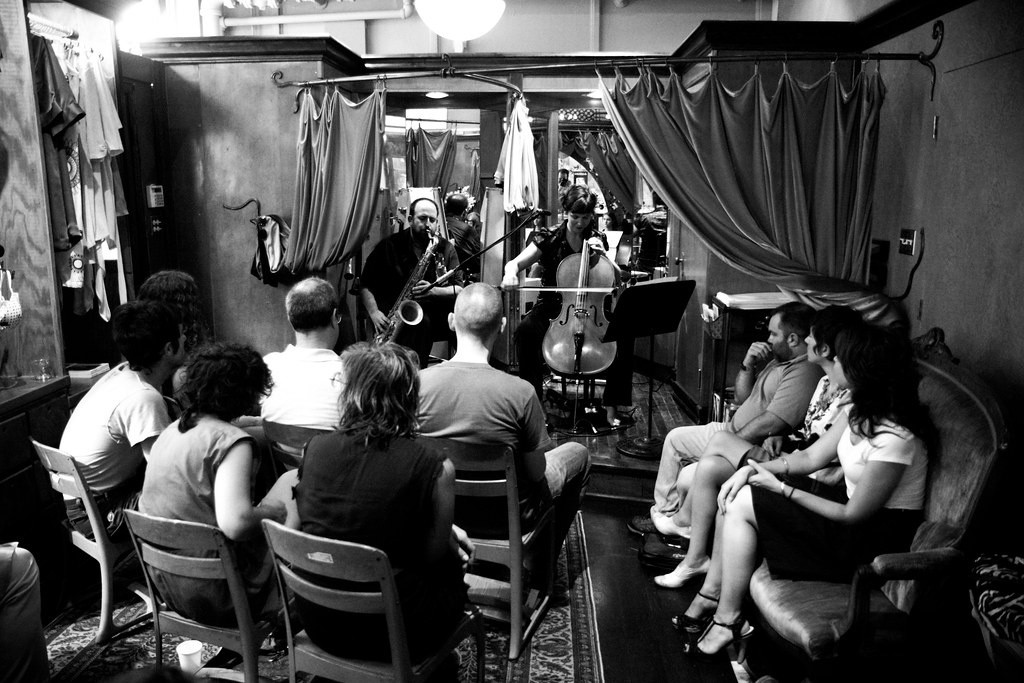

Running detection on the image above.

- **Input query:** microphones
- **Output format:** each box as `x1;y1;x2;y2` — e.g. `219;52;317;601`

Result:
535;208;552;217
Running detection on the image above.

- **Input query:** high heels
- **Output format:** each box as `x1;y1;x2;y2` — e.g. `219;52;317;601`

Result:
684;615;754;663
655;556;712;588
652;511;691;540
671;590;716;634
546;388;572;411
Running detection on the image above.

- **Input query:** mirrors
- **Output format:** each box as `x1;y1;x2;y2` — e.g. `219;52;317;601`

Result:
406;106;483;235
556;105;641;234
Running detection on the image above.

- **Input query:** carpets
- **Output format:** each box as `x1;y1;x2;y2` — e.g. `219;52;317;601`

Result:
40;509;609;683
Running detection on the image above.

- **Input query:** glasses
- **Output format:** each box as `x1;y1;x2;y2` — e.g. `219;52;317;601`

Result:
336;311;342;324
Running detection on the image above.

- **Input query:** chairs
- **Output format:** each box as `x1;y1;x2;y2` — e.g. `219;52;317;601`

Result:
28;394;563;683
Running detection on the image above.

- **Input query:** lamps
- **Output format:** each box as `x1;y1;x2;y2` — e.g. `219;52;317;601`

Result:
415;0;507;42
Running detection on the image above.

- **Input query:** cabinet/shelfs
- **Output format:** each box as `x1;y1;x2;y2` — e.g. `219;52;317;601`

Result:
707;293;773;422
0;363;114;626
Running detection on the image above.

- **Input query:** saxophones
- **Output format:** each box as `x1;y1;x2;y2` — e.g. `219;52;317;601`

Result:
373;229;441;349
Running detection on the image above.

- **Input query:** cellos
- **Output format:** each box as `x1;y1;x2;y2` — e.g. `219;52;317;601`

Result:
540;217;621;434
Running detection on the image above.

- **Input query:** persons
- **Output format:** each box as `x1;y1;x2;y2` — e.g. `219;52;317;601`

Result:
57;196;590;663
630;303;937;663
0;542;50;683
444;169;574;286
495;186;633;425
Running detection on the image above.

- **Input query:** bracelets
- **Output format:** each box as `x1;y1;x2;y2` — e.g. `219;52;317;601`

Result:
742;364;754;371
781;482;785;496
778;457;789;474
789;487;796;497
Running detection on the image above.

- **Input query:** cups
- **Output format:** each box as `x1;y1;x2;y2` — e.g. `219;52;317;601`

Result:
176;640;202;674
31;359;53;380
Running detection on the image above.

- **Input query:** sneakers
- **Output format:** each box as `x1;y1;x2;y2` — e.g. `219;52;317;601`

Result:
627;514;654;534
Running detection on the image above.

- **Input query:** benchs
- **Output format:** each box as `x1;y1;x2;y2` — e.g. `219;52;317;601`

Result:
707;328;1009;683
